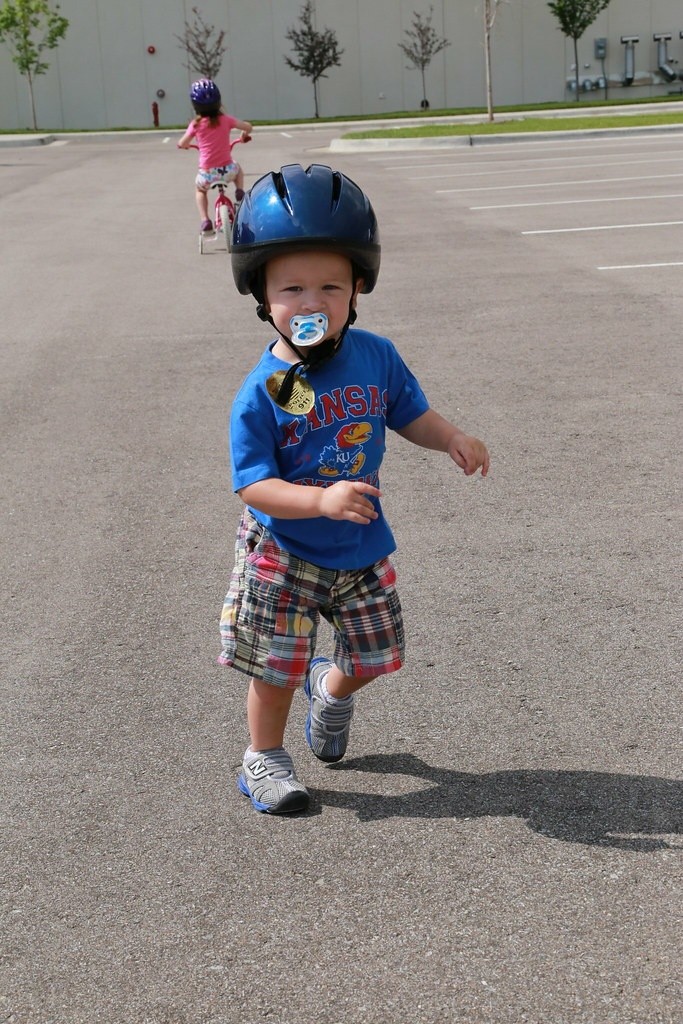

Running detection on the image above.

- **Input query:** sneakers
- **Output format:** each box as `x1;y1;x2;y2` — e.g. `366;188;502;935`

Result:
202;220;214;237
234;190;245;203
302;658;354;762
240;745;311;815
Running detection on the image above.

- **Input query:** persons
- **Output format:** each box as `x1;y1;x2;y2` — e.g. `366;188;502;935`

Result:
177;79;253;235
215;162;492;818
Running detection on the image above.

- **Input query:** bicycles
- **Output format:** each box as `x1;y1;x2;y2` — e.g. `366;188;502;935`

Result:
177;136;254;256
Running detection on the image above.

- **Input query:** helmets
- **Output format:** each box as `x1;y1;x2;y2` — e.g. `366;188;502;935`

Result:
190;79;222;115
231;163;382;295
